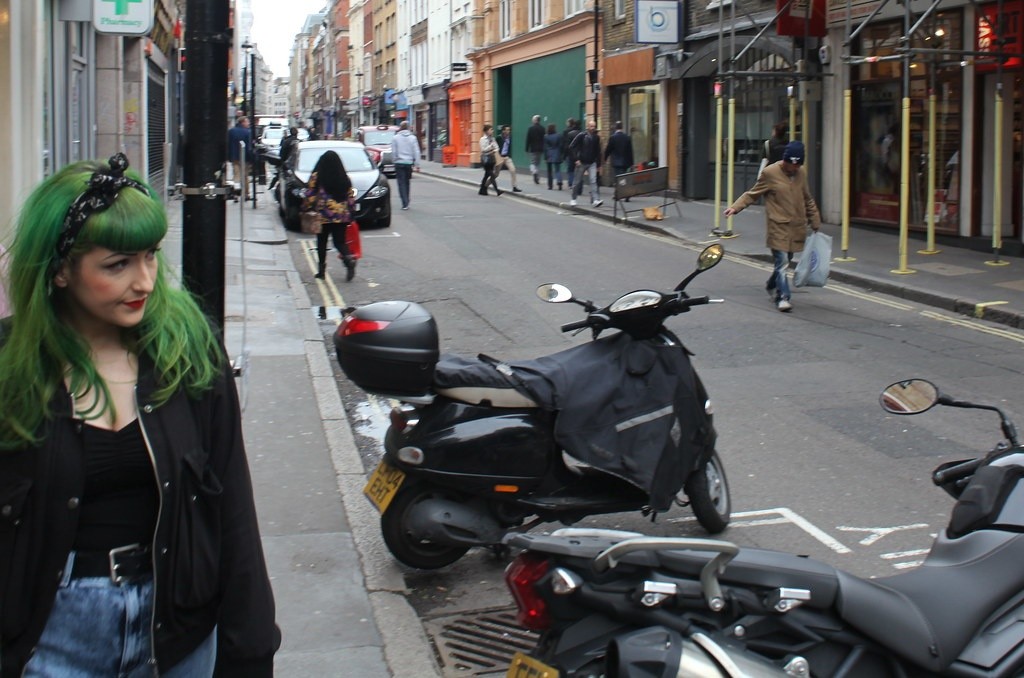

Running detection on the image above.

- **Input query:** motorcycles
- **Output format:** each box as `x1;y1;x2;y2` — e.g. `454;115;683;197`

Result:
502;378;1024;678
333;244;735;570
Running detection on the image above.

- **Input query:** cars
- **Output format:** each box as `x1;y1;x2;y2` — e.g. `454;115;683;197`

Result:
357;125;414;177
287;127;311;141
275;140;391;229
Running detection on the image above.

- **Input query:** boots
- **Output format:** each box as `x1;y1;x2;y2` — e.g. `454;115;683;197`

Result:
315;263;326;279
344;256;356;281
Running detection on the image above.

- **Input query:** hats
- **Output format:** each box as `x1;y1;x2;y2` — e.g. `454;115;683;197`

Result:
784;141;805;165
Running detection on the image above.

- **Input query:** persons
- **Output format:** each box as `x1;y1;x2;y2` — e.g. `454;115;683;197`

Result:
0;151;281;678
391;121;422;210
300;151;357;280
479;115;634;208
762;122;790;166
227;109;319;202
723;141;821;311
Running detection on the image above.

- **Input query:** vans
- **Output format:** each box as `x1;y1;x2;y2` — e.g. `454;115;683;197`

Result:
260;126;292;158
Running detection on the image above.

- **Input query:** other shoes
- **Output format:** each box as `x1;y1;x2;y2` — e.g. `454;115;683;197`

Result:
766;287;777;301
513;187;522;192
570;200;578;206
592;200;603;208
497;190;504;196
777;300;793;311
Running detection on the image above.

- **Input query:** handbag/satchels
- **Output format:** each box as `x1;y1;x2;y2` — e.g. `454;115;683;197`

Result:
337;221;361;260
792;231;834;287
757;158;769;180
302;211;322;234
481;154;495;167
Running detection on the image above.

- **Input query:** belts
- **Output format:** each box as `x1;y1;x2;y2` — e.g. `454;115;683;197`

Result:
72;544;152;583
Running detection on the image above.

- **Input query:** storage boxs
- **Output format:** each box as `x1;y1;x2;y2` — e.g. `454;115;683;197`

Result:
332;301;441;398
